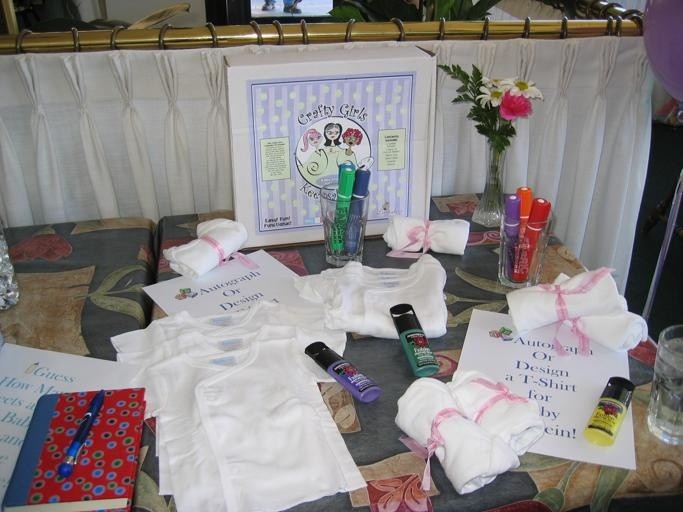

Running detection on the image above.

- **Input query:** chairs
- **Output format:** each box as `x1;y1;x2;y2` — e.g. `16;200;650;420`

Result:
641;1;683;327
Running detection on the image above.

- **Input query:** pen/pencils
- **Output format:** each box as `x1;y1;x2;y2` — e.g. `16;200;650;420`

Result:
58;390;104;477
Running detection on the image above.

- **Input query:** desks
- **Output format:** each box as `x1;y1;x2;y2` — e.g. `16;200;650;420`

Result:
0;217;154;511
132;193;682;512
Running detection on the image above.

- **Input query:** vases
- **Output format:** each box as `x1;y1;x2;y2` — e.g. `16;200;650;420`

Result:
473;143;508;227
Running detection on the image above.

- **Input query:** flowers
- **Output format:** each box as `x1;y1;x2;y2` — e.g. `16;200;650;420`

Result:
439;63;543;203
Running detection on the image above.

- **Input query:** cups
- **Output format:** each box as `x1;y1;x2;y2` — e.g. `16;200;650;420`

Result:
320;184;371;268
497;205;554;289
646;324;683;446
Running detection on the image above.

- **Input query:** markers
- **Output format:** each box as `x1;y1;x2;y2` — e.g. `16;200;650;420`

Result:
331;160;370;252
505;186;551;282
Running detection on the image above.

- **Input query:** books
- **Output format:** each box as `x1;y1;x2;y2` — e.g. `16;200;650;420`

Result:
2;388;146;512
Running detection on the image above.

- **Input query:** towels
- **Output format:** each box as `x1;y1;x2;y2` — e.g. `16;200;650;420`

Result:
163;216;247;279
382;211;470;257
395;376;519;497
506;268;618;332
553;274;647;353
446;373;545;455
